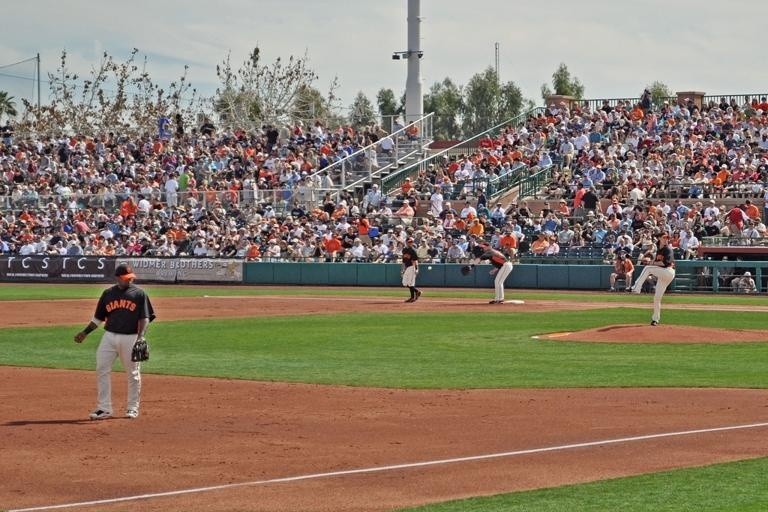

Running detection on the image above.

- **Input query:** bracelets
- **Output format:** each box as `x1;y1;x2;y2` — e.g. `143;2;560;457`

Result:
82;326;93;334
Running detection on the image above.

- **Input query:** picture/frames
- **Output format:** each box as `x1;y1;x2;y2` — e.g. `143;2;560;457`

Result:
346;216;423;228
519;216;583;226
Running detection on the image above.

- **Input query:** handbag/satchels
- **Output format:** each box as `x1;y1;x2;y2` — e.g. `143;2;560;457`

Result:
82;326;93;334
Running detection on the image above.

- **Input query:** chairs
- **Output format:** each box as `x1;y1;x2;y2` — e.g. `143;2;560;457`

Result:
651;321;659;327
125;409;139;419
625;286;640;294
489;299;504;305
405;298;416;302
415;290;422;300
89;409;112;419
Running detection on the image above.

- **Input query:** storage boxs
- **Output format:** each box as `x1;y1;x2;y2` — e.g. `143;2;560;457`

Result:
300;134;465;200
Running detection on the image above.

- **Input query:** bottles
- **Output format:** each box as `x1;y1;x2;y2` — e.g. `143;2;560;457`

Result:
407;237;413;242
115;264;136;281
659;232;671;240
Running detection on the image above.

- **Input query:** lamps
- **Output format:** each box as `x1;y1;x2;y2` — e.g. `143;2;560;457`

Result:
380;223;395;234
443;226;685;294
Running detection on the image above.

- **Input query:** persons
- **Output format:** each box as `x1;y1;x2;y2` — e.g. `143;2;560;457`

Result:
624;232;675;325
73;264;156;421
276;119;518;264
401;237;421;303
511;90;766;294
462;242;513;304
1;114;280;262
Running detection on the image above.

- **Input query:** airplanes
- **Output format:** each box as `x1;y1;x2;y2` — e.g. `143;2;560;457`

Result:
461;266;472;275
131;340;149;361
642;258;653;266
